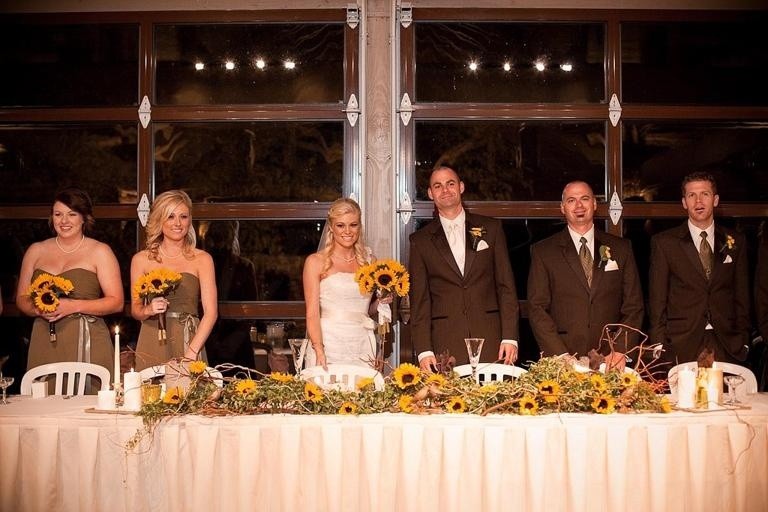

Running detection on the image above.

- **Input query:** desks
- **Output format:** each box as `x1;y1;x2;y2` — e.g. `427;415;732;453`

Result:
0;391;768;512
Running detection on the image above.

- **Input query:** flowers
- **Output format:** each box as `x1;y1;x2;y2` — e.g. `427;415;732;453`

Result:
19;273;74;342
354;259;411;336
133;267;182;341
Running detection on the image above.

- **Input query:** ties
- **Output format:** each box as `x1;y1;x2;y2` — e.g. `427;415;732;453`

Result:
579;238;593;289
449;224;463;276
700;232;714;280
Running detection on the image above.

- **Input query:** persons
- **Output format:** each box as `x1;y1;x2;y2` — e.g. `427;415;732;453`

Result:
407;166;520;375
526;180;645;368
196;219;259;379
755;219;768;344
645;170;750;394
130;190;219;371
16;190;125;395
295;197;395;393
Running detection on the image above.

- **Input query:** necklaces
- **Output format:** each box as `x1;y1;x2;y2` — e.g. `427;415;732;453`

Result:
159;245;183;259
334;252;357;264
56;235;85;253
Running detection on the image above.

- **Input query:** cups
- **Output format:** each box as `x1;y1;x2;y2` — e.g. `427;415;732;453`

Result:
140;384;162;406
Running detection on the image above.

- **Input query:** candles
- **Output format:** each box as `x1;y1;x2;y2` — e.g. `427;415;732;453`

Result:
701;385;718;408
114;325;120;383
678;366;696;408
706;361;723;408
124;368;141;410
97;391;116;410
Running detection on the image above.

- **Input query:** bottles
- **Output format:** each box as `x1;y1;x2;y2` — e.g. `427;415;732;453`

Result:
250;326;258;342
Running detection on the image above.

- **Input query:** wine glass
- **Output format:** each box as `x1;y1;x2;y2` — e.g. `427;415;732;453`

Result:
288;339;308;380
723;376;745;404
463;338;485;384
0;376;14;404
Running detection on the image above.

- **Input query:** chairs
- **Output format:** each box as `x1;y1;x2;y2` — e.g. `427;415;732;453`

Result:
572;363;643;383
668;361;758;394
139;366;223;389
20;361;111;395
295;364;385;392
453;363;528;384
